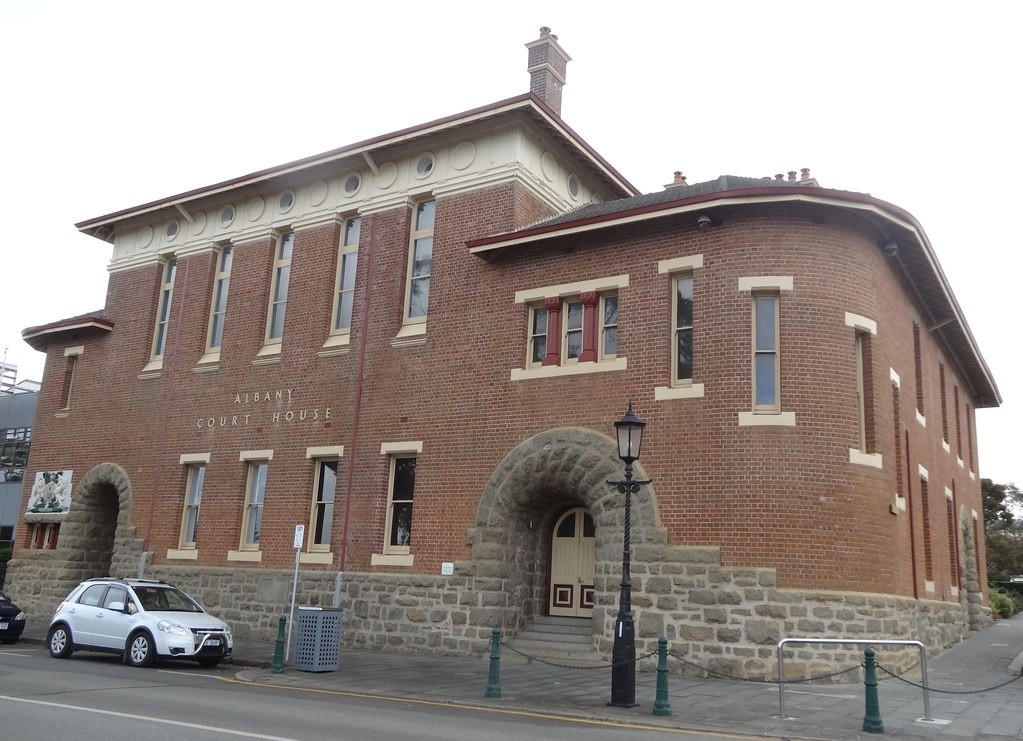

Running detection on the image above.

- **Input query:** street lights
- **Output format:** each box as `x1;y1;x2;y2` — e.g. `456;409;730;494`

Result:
606;398;653;708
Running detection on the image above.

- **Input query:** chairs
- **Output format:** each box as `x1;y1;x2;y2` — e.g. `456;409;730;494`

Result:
143;592;158;608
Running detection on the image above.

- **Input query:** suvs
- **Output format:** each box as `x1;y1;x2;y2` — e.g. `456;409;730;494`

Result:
46;577;233;668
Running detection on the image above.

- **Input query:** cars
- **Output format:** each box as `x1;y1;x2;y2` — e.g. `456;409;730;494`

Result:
0;590;26;644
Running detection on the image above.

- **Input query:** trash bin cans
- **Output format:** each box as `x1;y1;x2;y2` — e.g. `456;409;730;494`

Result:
291;606;343;673
